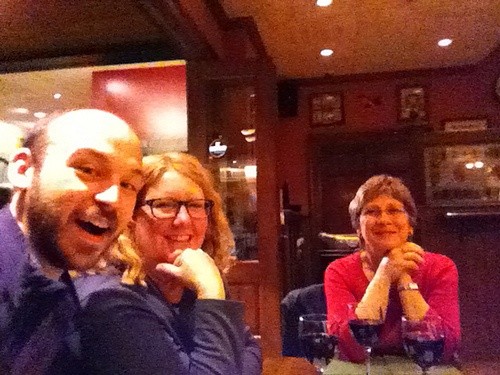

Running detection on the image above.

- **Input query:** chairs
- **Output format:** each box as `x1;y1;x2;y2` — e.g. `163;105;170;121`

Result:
281;284;326;357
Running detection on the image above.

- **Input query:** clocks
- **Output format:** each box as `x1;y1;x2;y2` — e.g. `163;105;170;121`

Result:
307;90;345;128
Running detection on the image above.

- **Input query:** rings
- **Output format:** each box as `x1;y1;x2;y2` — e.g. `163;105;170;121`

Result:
399;246;405;253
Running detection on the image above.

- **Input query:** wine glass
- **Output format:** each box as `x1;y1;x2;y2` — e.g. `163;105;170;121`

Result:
347;302;385;375
401;315;446;375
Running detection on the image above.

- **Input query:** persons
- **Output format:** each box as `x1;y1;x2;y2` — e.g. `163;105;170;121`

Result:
322;174;462;363
72;152;262;375
0;106;147;375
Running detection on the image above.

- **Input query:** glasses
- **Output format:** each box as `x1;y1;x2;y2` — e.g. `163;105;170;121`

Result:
360;208;408;217
133;198;216;220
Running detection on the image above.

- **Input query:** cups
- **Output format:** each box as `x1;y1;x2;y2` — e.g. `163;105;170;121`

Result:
298;314;339;375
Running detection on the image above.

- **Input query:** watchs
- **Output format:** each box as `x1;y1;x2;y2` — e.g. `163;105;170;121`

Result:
398;282;418;292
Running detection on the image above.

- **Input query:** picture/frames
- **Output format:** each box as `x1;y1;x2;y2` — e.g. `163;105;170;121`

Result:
395;82;431;122
